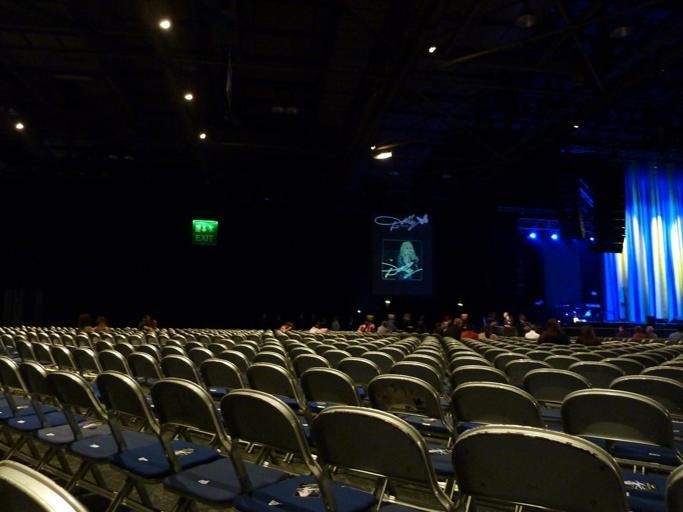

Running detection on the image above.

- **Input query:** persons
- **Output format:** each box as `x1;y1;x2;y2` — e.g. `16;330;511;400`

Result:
438;311;541;340
396;241;421;281
309;323;329;334
75;314;93;335
357;314;415;334
92;315;110;333
574;325;602;347
137;315;153;331
150;319;159;328
616;326;657;339
537;322;571;345
279;321;294;333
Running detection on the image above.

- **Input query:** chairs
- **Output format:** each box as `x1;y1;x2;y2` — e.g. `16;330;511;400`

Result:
1;316;681;512
